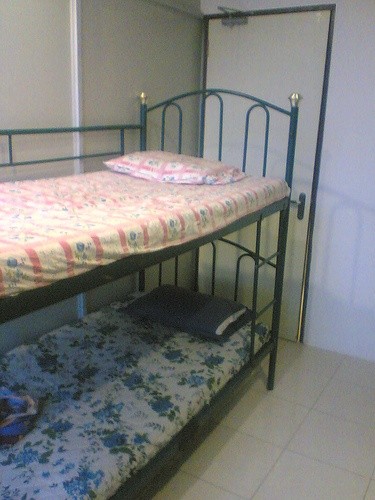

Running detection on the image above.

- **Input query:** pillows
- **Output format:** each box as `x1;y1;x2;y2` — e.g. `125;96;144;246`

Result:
116;284;261;346
101;150;248;185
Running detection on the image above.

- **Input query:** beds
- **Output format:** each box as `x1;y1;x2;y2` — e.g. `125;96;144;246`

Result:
0;88;302;499
